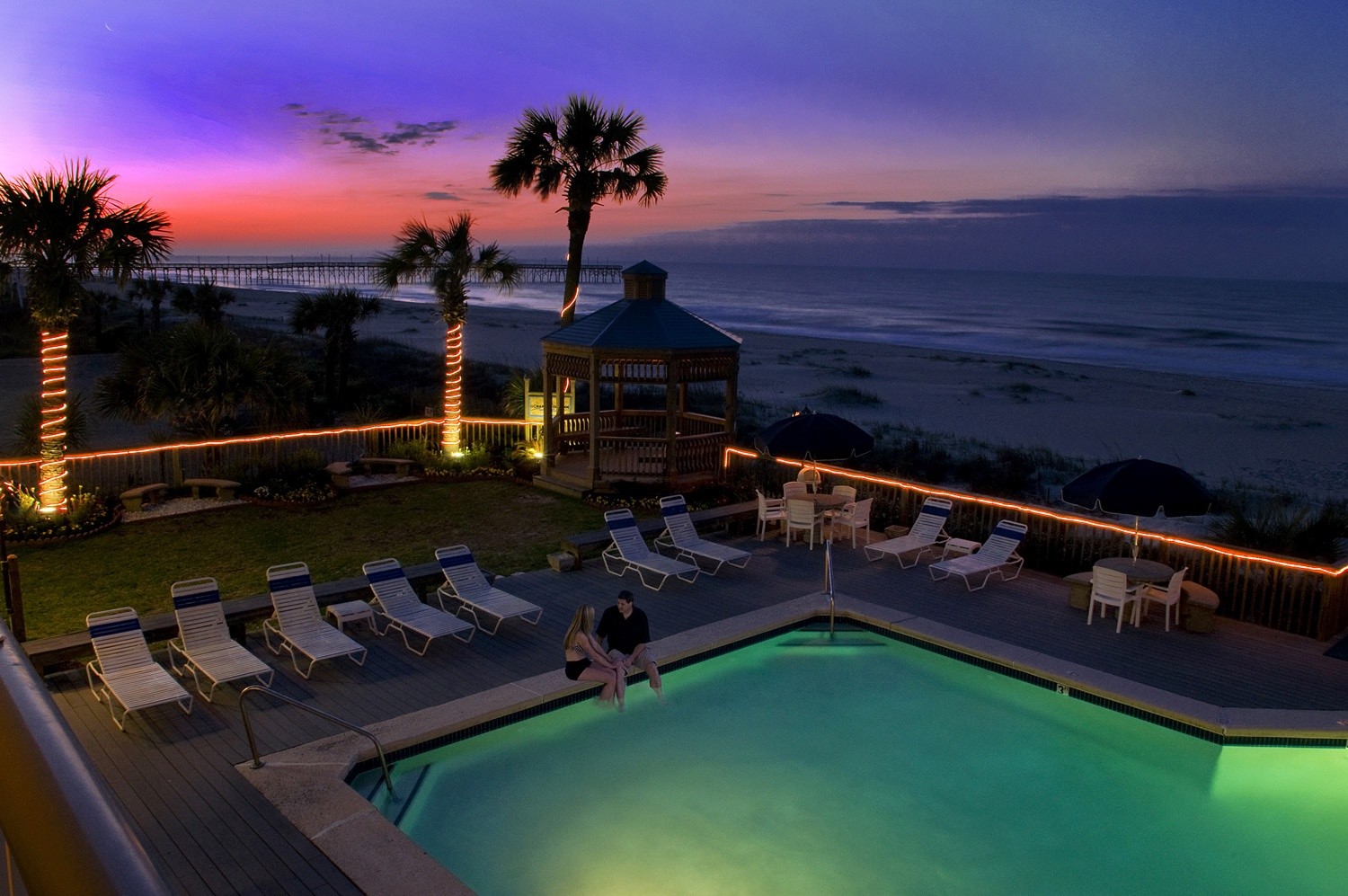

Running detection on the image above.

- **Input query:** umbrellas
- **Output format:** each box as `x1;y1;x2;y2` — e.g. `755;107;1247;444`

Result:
753;410;876;495
1060;455;1229;561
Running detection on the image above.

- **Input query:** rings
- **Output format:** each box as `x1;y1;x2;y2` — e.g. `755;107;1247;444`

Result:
620;658;621;659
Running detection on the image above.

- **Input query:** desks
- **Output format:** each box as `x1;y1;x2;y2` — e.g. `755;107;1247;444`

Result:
789;494;847;543
1096;558;1174;624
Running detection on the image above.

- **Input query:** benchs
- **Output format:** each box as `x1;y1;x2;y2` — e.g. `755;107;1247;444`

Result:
120;479;241;511
638;452;721;475
1063;572;1220;635
325;457;414;487
557;427;642;454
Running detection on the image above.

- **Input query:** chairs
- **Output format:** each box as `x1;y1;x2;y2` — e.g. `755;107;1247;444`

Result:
928;520;1028;592
755;481;874;550
87;493;750;733
1134;566;1189;631
1088;566;1137;633
863;497;953;569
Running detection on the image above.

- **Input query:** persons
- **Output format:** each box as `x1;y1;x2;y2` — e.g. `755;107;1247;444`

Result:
563;603;624;701
596;590;661;713
